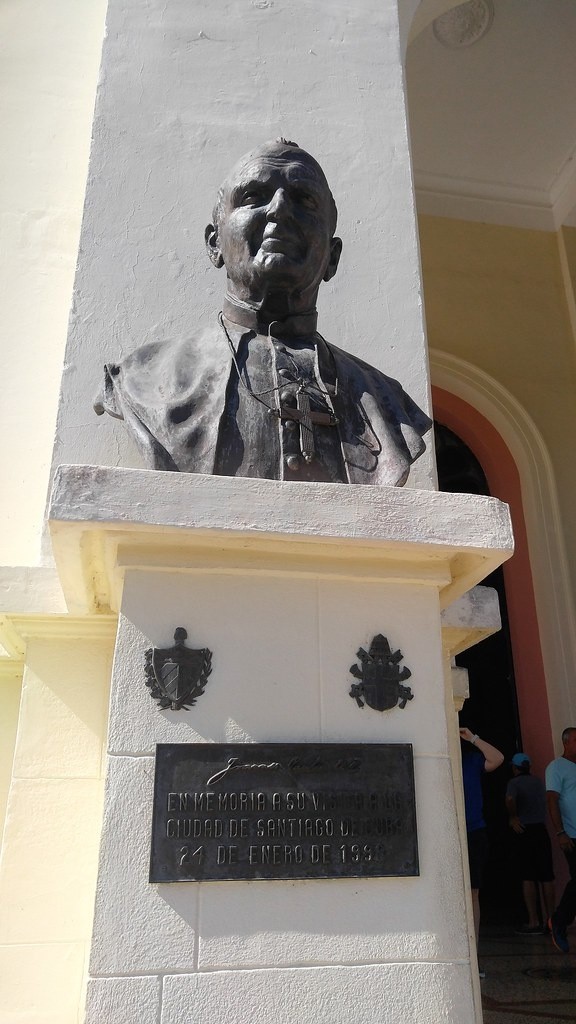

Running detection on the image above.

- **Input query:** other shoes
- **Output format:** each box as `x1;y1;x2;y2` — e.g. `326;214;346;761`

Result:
513;924;544;935
479;969;486;978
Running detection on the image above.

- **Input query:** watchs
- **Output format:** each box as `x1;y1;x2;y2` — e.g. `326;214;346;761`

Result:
472;734;479;742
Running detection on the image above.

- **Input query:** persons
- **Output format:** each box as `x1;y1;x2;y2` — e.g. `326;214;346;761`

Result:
505;752;556;936
545;728;576;954
94;135;434;485
459;727;505;978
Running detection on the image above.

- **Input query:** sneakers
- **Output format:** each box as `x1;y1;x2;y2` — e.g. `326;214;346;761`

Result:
547;917;570;954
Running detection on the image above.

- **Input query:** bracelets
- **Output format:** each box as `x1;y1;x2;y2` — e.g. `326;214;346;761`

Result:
557;829;566;835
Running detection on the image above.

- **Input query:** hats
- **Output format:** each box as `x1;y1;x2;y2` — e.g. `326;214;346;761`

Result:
511;753;531;770
